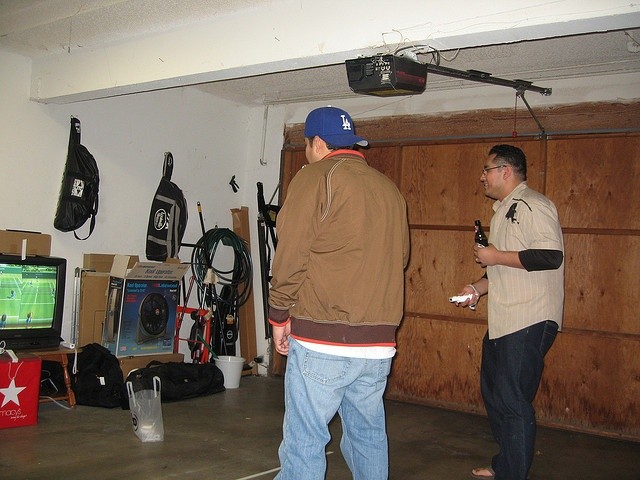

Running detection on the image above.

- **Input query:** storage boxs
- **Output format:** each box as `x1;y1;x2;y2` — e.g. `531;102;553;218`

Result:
99;254;193;360
83;253;141;271
78;271;110;349
118;352;186;383
230;205;259;375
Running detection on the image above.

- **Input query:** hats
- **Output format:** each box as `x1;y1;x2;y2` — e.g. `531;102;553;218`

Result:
304;105;369;148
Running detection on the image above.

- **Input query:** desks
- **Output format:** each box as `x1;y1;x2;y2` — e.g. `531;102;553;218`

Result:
1;342;84;409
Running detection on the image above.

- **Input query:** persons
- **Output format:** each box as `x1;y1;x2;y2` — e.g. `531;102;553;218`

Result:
266;105;411;480
452;143;568;480
25;312;32;329
0;314;7;329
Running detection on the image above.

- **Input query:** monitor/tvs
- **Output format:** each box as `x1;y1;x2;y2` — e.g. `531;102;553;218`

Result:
0;253;67;350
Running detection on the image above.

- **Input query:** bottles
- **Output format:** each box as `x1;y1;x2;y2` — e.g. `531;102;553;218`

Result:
475;219;487;248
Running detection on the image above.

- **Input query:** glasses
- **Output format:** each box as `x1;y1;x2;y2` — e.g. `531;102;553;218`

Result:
482;165;507;174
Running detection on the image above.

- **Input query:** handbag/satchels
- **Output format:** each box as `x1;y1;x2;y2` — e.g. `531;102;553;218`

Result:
54;117;99;232
146;153;188;262
127;376;164;443
123;360;226;409
67;343;124;408
40;360;67;397
0;353;42;429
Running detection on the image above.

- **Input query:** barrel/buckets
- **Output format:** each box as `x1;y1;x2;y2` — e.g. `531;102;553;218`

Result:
212;356;245;389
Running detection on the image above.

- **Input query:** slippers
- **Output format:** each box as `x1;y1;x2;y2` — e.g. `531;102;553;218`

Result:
468;466;495;479
